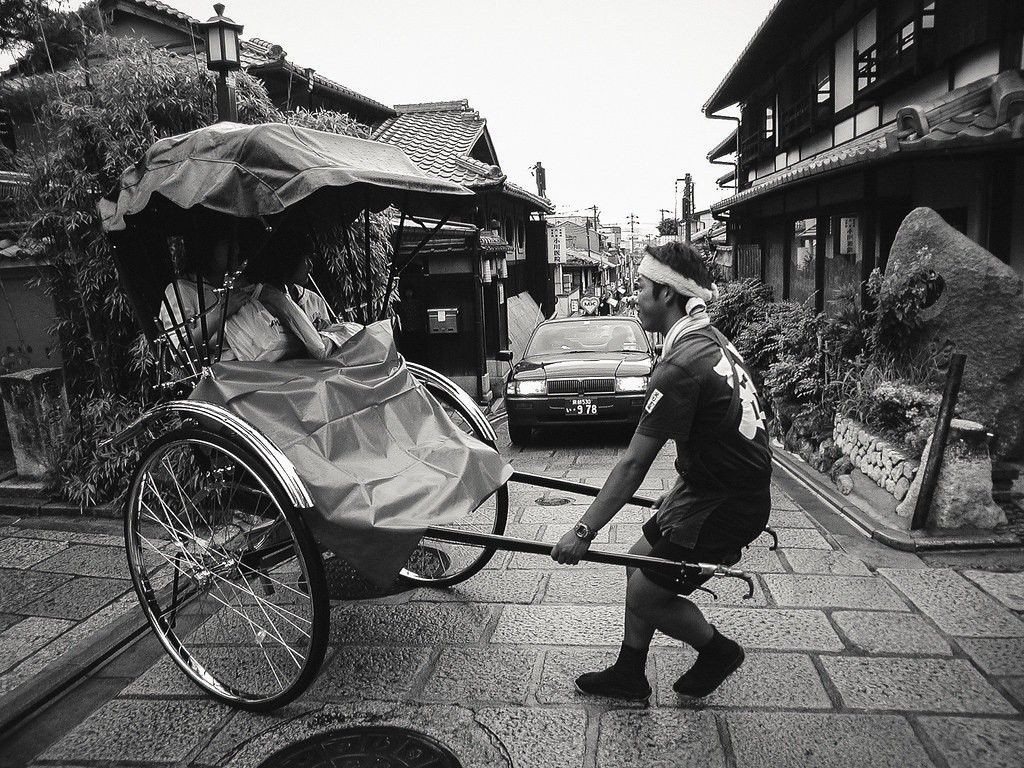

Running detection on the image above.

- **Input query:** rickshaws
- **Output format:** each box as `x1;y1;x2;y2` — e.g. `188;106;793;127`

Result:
94;119;781;713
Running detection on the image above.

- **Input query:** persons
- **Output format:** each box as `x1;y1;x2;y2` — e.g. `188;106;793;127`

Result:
152;209;345;384
623;297;640;315
548;239;778;701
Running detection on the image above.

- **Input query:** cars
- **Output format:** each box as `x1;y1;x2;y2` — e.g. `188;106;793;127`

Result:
494;313;662;448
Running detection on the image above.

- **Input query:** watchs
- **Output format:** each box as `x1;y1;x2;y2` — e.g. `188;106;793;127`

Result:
574;521;598;541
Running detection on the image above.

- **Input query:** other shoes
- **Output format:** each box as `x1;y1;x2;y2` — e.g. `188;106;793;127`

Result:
671;639;745;698
573;668;652;701
